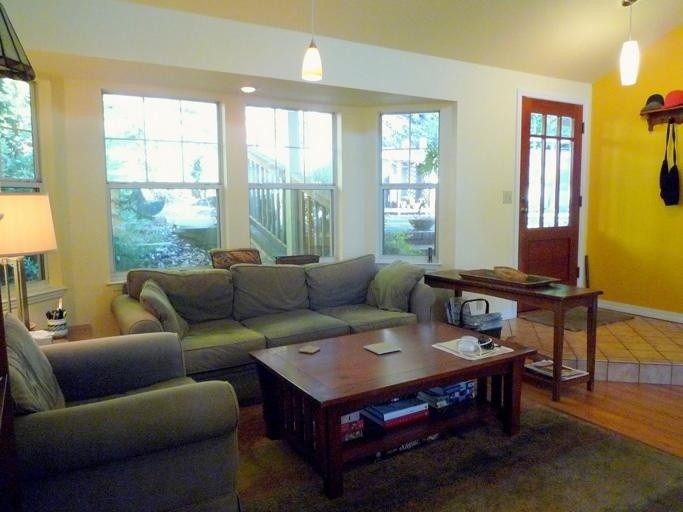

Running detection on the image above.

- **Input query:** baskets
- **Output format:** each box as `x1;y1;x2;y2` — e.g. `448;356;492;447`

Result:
448;299;502;340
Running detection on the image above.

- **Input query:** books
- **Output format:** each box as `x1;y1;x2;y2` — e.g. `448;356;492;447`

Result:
362;397;429;429
417;380;475;409
282;388;366;441
526;360;590;382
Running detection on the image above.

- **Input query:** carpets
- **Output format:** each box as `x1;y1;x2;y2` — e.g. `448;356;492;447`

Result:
225;396;683;511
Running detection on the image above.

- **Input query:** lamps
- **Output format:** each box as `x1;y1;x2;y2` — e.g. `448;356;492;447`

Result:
618;0;639;86
301;0;323;82
0;194;60;332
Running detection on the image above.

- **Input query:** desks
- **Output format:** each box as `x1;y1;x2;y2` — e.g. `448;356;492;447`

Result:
423;262;603;402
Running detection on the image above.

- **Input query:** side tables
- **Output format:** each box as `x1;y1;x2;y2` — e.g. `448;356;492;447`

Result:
31;320;93;346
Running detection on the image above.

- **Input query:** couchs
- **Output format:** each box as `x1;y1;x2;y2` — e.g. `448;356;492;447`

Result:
0;313;241;512
109;254;450;408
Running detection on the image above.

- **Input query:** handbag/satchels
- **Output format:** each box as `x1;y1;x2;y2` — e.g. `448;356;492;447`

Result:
660;117;680;206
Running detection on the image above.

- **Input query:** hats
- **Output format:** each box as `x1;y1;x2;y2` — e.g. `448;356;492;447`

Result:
641;101;662;111
646;94;664;106
664;90;683;109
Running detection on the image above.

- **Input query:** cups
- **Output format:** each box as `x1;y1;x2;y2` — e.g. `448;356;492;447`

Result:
47;318;68;340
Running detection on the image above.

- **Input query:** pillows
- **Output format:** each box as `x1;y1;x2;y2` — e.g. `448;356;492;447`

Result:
305;253;375;311
231;263;309;320
126;267;233;322
143;281;189;345
365;259;422;312
